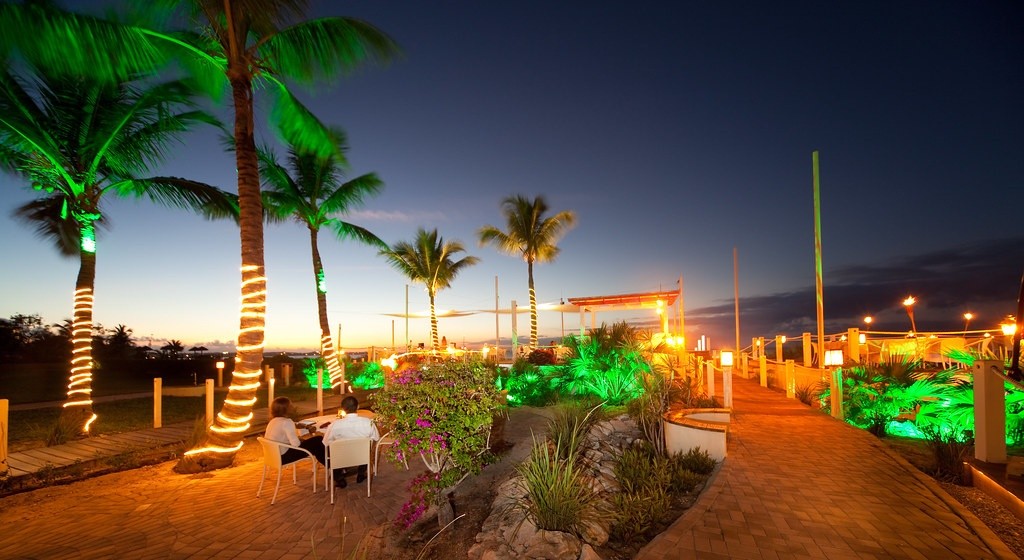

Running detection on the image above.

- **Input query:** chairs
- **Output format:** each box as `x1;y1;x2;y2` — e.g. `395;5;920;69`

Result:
325;438;371;503
256;436;317;505
372;429;409;477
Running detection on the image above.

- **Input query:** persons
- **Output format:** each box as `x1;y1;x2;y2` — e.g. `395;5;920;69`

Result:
264;397;331;468
321;396;381;489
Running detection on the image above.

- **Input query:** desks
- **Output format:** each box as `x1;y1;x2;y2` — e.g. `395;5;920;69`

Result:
295;414;374;433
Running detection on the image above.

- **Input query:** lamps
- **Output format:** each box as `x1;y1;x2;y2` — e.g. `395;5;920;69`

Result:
825;350;843;366
720;352;733;366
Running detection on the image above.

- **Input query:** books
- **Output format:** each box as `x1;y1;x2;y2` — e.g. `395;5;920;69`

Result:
298;419;317;426
